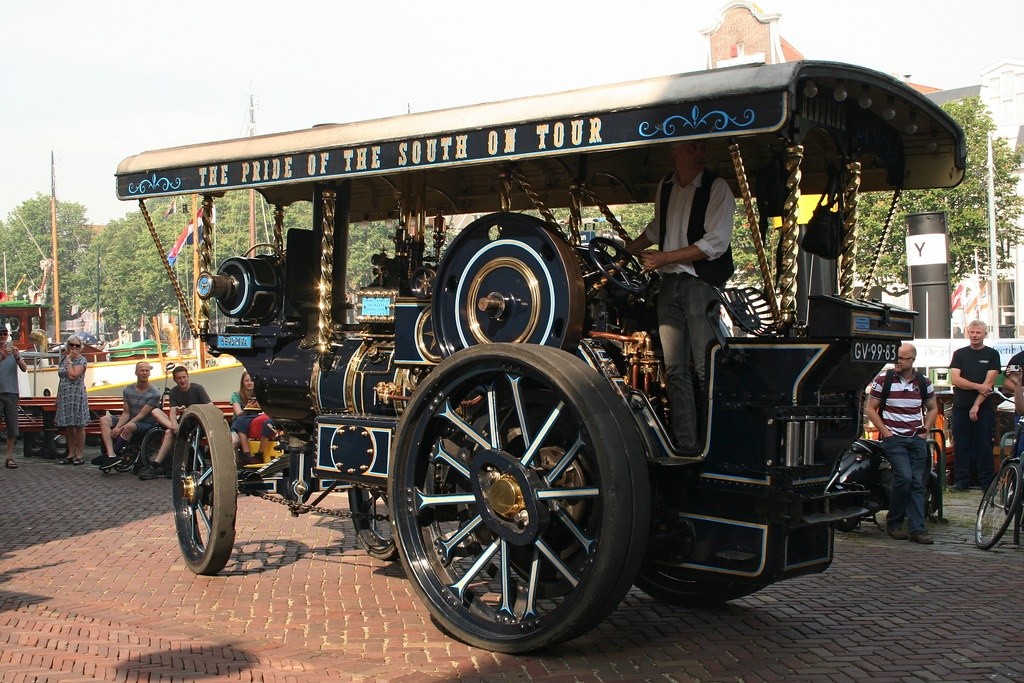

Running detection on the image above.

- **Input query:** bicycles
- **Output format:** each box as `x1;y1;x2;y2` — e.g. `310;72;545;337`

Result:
973;387;1024;550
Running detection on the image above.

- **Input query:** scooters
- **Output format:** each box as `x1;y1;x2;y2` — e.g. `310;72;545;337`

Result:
837;434;939;532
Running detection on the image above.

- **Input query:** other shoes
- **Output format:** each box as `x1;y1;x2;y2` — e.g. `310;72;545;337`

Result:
948;484;970;493
887;523;909;540
909;531;935;545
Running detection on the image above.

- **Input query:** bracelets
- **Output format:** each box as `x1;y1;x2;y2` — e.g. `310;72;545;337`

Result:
16;356;21;361
68;362;73;364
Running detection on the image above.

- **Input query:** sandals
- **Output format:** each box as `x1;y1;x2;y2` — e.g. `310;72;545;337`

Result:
5;459;18;469
59;456;76;465
73;456;87;465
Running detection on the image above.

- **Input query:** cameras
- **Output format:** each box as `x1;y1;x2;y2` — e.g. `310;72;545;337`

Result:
1009;365;1019;372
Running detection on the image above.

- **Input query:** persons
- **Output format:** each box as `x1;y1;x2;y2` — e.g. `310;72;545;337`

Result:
625;139;735;452
138;366;212;479
0;326;27;469
865;343;939;545
53;335;90;465
1003;351;1024;457
230;371;276;463
100;362;160;469
949;320;1002;496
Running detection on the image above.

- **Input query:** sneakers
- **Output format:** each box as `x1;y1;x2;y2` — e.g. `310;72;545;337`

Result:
98;455;122;470
139;466;158;480
91;454;109;466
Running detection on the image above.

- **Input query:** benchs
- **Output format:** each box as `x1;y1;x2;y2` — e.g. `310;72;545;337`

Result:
0;414;102;434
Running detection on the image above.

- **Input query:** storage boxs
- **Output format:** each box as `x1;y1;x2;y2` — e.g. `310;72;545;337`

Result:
808;294;919;340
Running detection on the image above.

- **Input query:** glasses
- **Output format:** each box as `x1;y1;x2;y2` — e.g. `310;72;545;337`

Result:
0;333;8;336
67;342;81;348
898;356;913;361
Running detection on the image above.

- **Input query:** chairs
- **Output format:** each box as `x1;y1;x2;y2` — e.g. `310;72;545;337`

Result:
710;285;776;337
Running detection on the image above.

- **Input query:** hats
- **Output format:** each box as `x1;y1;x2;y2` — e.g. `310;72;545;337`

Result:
0;325;9;332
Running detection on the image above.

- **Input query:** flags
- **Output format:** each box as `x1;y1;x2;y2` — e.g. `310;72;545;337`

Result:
165;200;174;217
167;206;204;268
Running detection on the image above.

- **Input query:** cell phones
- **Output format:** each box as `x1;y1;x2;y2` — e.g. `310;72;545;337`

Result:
7;341;13;353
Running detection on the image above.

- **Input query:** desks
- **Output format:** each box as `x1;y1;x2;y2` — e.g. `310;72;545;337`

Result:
18;396;234;457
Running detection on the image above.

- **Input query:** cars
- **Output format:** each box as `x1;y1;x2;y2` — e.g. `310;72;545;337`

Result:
115;61;965;659
